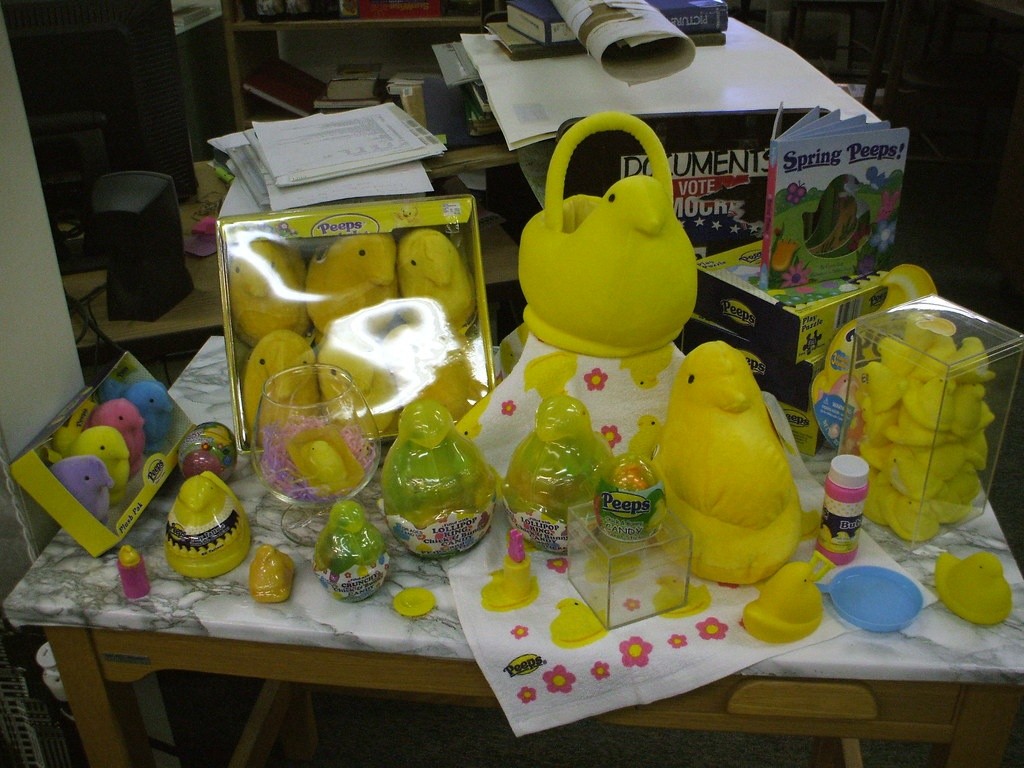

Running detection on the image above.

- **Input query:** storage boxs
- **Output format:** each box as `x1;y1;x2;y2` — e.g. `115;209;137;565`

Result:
780;403;826;457
214;172;497;453
694;239;889;363
10;352;197;556
835;293;1024;552
672;312;826;413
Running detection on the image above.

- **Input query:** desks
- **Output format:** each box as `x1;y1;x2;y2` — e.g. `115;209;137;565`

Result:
41;159;520;346
2;335;1024;768
878;0;1024;319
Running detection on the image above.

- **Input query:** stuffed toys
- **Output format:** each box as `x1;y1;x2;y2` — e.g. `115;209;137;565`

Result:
517;114;697;358
10;228;1010;642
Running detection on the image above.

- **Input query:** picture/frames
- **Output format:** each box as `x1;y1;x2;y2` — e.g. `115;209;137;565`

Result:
771;12;851;76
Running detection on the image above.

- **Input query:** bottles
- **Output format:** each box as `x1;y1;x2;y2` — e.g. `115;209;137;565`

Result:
814;453;870;567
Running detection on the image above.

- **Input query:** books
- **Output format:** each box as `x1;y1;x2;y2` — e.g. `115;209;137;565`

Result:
431;0;728;136
207;57;506;213
761;101;910;291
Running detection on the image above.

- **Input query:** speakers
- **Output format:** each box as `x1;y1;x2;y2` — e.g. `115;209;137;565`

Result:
92;171;194;324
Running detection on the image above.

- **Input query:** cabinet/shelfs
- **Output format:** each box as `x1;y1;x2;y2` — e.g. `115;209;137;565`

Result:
221;0;518;179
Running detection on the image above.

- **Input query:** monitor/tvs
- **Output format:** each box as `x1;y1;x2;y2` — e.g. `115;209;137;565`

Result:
1;0;198;276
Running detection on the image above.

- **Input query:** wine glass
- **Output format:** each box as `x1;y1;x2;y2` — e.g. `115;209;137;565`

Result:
250;362;382;549
